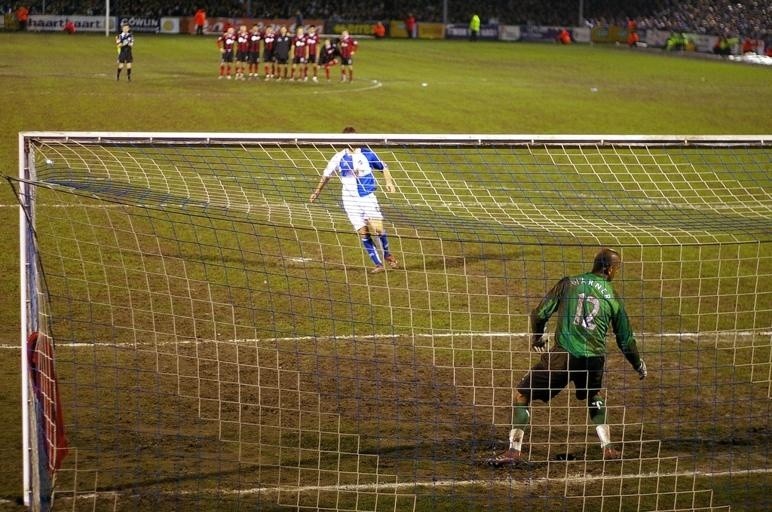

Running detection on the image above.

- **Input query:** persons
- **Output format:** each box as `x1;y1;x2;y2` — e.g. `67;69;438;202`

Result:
116;24;135;82
1;0;771;60
216;22;357;85
478;247;647;471
310;126;401;273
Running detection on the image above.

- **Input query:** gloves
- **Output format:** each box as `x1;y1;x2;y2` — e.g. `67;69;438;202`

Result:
634;357;649;381
531;334;547;353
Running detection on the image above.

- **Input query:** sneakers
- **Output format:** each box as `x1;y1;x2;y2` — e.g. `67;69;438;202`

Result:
371;266;386;273
602;447;625;460
384;253;401;269
216;73;354;85
476;449;525;469
